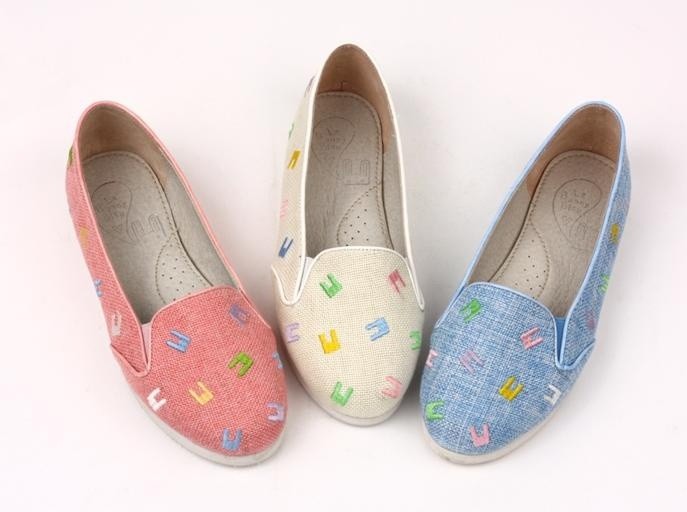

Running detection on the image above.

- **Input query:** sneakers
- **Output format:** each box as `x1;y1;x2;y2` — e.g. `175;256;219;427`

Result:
271;40;428;430
62;99;290;470
417;99;631;469
